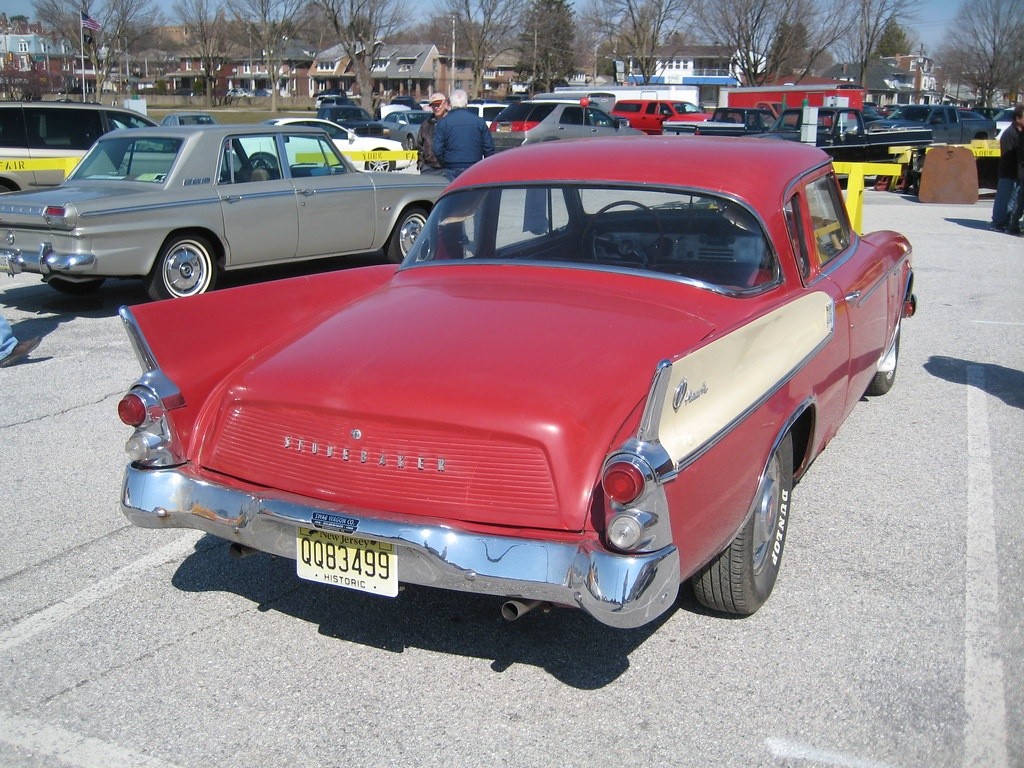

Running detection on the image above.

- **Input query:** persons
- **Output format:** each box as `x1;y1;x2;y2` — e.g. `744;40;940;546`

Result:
988;106;1024;235
432;90;495;257
417;93;449;176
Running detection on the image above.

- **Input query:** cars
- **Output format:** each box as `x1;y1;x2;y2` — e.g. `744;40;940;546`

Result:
251;89;271;96
466;94;508;130
160;113;218;127
377;110;438;152
862;101;1017;147
1;126;452;303
227;88;255;98
490;100;650;155
114;136;917;630
315;94;356;111
315;106;381;137
225;120;417;174
138;87;158;95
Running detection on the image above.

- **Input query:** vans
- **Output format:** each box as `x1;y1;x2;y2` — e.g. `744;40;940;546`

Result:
611;99;712;138
1;98;163;195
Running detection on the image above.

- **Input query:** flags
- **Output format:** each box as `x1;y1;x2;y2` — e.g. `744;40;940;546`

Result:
81;12;101;32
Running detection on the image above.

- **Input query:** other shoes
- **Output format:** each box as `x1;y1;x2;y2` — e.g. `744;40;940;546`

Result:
1009;228;1020;235
991;225;1002;232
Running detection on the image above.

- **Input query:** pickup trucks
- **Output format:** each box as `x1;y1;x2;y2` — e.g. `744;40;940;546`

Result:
754;106;933;163
661;108;793;136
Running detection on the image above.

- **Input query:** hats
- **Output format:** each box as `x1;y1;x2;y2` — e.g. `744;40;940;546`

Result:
429;93;446;107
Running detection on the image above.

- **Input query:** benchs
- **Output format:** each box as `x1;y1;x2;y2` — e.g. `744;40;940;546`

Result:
579;213;756;286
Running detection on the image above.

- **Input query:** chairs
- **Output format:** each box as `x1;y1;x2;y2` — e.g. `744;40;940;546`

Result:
239;152;279;181
726;117;736;123
